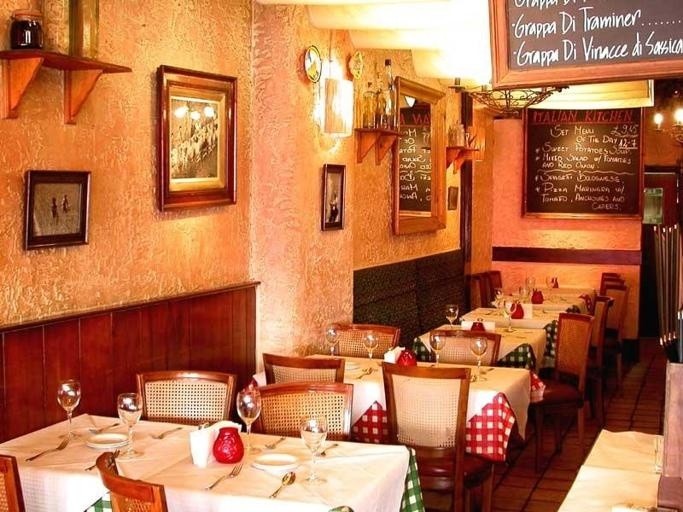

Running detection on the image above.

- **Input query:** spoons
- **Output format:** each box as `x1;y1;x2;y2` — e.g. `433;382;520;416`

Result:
269;471;295;498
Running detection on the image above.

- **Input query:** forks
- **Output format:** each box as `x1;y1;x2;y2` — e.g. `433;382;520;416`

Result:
25;435;70;461
203;463;242;491
88;423;119;434
84;449;120;471
264;435;286;449
315;443;337;456
356;368;374;380
151;427;182;439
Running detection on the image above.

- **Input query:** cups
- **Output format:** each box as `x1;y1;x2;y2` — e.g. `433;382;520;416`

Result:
653;433;667;475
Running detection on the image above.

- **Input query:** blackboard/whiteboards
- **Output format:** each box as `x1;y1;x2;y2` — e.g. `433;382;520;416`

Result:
399;106;431;217
488;0;683;91
520;107;643;220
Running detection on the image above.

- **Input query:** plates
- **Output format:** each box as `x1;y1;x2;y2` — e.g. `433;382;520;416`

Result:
85;433;128;449
344;360;362;370
250;454;301;471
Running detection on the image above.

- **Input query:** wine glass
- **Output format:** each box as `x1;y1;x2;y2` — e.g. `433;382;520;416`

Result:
56;379;81;438
323;321;342;360
428;272;556;381
236;391;262;454
300;414;328;485
117;393;143;458
362;330;379;369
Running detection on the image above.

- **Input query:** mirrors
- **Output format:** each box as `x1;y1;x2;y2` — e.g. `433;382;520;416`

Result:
391;76;447;236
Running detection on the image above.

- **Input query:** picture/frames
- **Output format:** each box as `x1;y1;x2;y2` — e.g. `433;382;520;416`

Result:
319;161;346;232
156;63;239;212
23;168;92;251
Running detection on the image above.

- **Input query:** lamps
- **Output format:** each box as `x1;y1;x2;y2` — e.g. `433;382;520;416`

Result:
653;109;683;144
322;75;353;155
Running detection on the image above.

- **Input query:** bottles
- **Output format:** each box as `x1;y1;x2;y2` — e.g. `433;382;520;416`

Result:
213;426;243;463
10;7;44;48
361;57;397;130
449;122;470;150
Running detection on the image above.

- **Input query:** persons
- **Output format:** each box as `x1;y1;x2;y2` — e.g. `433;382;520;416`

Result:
50;196;57;219
60;195;68;213
328;190;338;223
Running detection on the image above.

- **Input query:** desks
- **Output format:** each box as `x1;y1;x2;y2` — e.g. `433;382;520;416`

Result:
559;419;683;511
